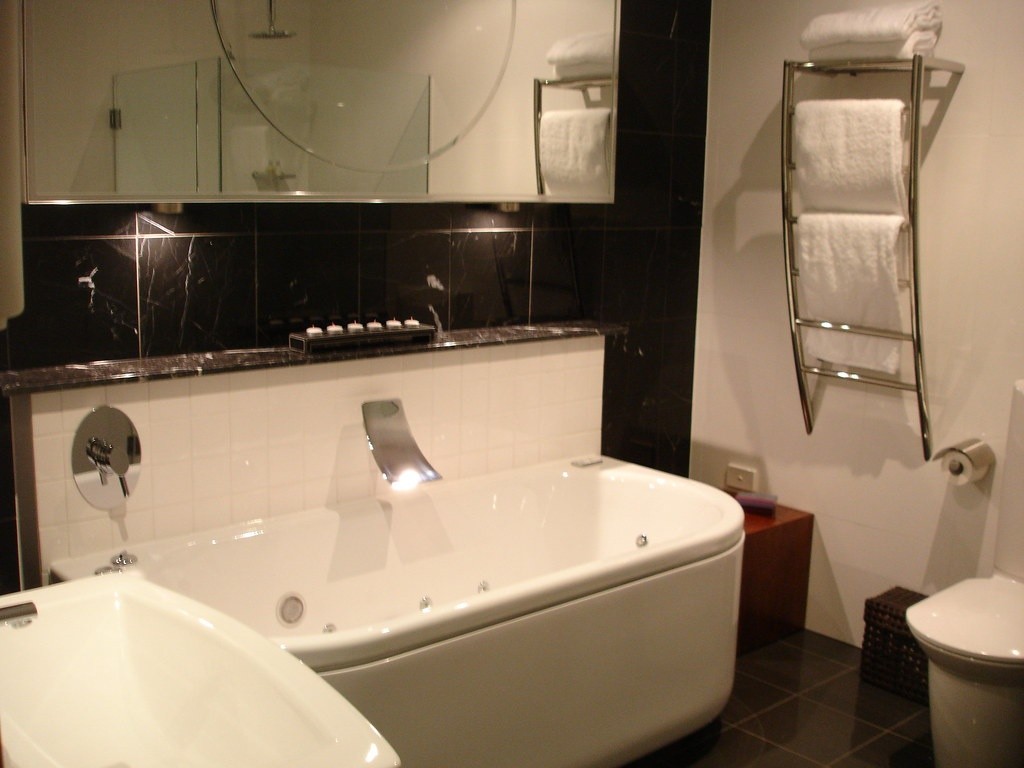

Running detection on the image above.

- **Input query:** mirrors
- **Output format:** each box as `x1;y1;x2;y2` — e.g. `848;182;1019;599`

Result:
16;0;620;204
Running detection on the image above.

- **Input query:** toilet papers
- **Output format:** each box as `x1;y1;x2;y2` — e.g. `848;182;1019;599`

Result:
942;445;991;486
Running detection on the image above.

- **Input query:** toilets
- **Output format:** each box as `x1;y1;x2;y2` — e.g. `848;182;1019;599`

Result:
905;380;1024;768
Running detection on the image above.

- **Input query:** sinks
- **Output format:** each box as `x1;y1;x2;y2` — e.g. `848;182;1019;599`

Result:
0;573;406;768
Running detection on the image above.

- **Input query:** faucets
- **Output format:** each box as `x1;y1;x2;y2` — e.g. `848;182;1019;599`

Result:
1;602;38;619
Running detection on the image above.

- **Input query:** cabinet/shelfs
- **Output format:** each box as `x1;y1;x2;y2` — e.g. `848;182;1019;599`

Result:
725;490;814;648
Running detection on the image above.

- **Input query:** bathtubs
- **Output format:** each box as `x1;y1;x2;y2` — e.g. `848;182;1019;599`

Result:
51;457;745;768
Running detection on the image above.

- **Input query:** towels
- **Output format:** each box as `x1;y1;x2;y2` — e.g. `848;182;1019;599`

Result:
538;108;612;201
548;32;619;78
799;4;941;62
799;216;910;375
791;98;908;214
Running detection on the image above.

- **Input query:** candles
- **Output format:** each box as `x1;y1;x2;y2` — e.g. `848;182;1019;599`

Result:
307;319;420;336
